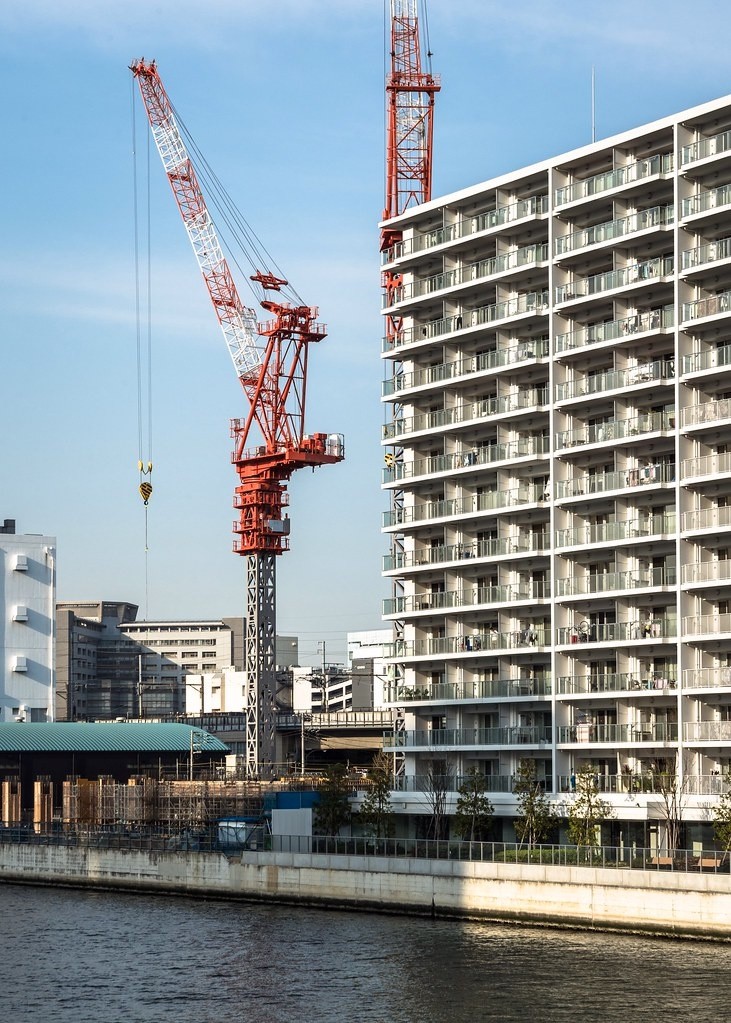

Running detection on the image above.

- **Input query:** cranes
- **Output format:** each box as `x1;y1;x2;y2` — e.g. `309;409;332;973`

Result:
129;54;349;848
384;1;442;787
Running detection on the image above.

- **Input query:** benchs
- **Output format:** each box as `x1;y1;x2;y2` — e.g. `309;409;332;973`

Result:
691;859;721;872
646;857;672;870
